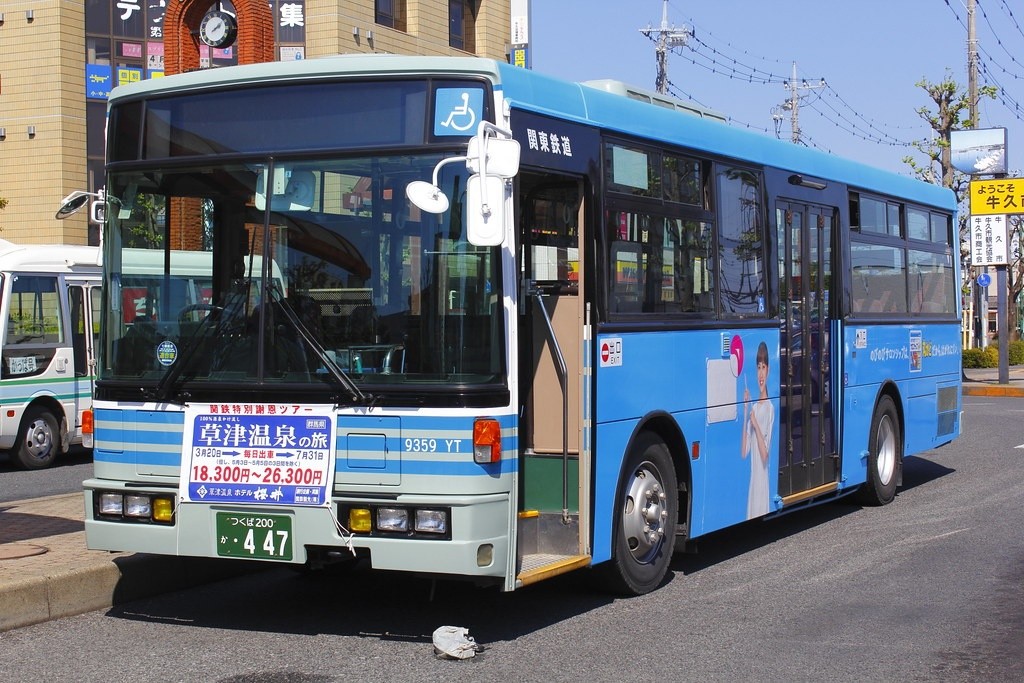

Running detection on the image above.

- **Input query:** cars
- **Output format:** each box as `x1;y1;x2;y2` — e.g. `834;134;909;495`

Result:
779;314;829;397
807;290;829;313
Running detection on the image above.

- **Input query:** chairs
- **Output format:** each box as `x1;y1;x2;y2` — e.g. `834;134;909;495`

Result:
248;295;378;371
852;271;946;313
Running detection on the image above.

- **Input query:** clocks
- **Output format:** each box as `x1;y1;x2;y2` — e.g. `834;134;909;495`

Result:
199;10;238;49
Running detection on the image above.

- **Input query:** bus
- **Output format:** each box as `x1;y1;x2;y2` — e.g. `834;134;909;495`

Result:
55;53;964;596
0;239;287;472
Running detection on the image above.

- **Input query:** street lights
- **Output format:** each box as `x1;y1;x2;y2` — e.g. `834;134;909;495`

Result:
968;268;976;350
